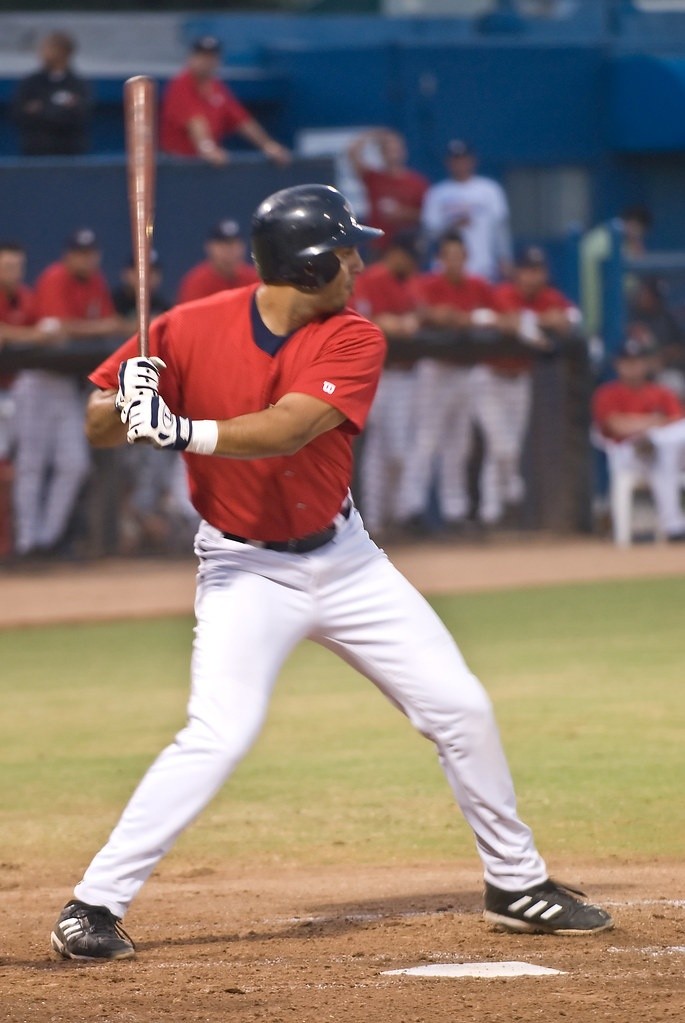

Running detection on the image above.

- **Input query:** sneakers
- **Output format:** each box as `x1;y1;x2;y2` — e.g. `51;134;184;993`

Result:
49;902;138;963
483;876;612;937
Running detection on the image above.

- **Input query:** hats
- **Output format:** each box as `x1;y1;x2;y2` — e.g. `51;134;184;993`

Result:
521;246;547;269
193;38;221;54
68;228;99;252
209;217;246;243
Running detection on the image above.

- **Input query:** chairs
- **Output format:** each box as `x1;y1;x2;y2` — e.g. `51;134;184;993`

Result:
590;365;684;546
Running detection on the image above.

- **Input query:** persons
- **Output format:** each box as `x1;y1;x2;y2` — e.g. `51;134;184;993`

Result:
160;35;291;166
109;251;174;556
347;234;581;536
421;143;518;283
0;225;121;564
15;31;88;153
590;209;685;537
350;132;428;250
50;185;614;958
174;219;260;538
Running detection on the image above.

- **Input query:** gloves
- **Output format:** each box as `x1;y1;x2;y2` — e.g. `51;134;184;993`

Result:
118;386;219;458
114;354;167;412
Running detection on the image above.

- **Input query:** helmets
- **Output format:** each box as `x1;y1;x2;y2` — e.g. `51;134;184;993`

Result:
250;185;384;292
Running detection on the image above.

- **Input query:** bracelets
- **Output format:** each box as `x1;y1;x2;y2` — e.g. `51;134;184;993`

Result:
267;142;281;152
200;140;214;151
185;419;218;455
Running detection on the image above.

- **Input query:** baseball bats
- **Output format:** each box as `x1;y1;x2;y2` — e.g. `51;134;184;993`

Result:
123;75;157;443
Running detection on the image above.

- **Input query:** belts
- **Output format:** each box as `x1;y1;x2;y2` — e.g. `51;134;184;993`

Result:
225;499;354;552
